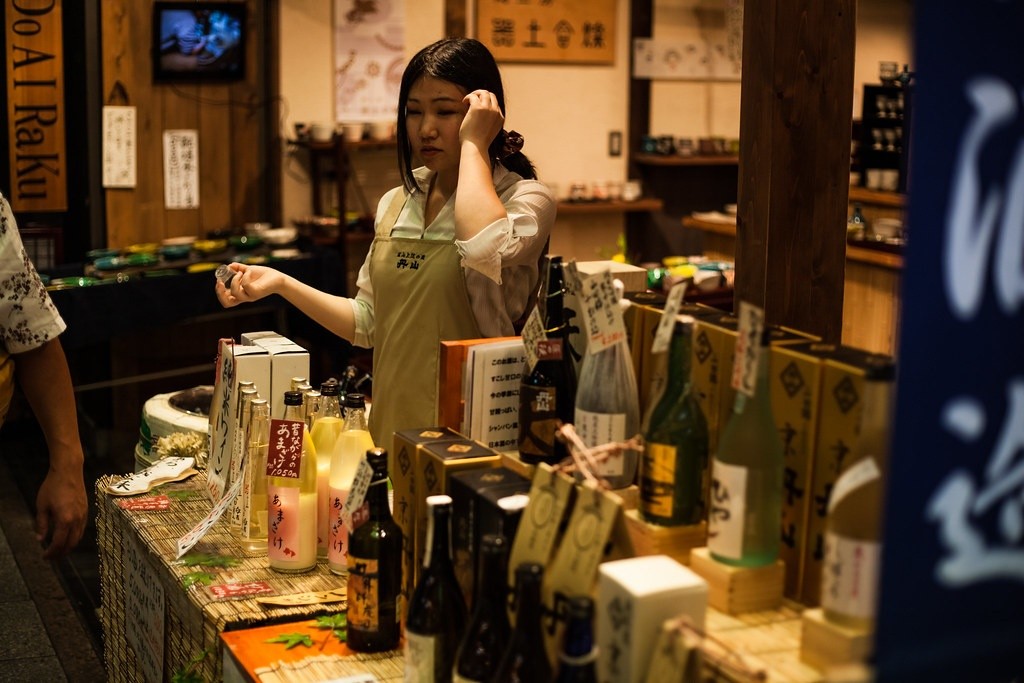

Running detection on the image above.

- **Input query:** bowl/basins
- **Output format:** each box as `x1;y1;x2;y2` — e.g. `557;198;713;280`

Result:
39;221;300;289
872;218;902;237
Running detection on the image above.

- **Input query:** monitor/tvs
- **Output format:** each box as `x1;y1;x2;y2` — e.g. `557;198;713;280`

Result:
151;0;250;85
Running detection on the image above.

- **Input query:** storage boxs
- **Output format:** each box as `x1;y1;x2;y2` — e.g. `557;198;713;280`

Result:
448;467;530;589
621;290;670;388
769;341;870;603
258;344;310;419
715;324;822;454
206;345;271;505
412;440;501;591
688;312;781;522
240;331;282;345
639;302;718;420
250;336;296;347
392;426;467;594
595;555;708;683
475;482;533;595
799;354;895;609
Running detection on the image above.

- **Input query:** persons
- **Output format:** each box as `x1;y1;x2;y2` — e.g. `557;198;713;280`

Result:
0;192;88;561
217;38;555;466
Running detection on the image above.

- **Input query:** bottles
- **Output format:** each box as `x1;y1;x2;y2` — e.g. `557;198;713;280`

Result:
850;208;864;224
637;314;705;526
224;376;374;577
573;278;637;492
548;178;642;203
518;253;573;462
346;446;405;651
642;135;737;155
708;321;783;568
879;62;898;87
820;354;895;630
404;495;466;683
494;562;554;682
551;597;599;682
449;534;514;682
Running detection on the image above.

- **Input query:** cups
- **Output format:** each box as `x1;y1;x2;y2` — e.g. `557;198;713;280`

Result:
309;122;398;143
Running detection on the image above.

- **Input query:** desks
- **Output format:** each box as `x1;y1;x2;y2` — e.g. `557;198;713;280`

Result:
95;445;878;683
